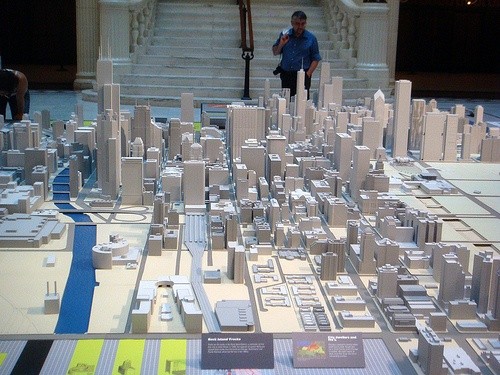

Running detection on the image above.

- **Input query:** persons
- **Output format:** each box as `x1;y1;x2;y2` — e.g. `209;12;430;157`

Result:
272;10;321;101
0;66;30;122
